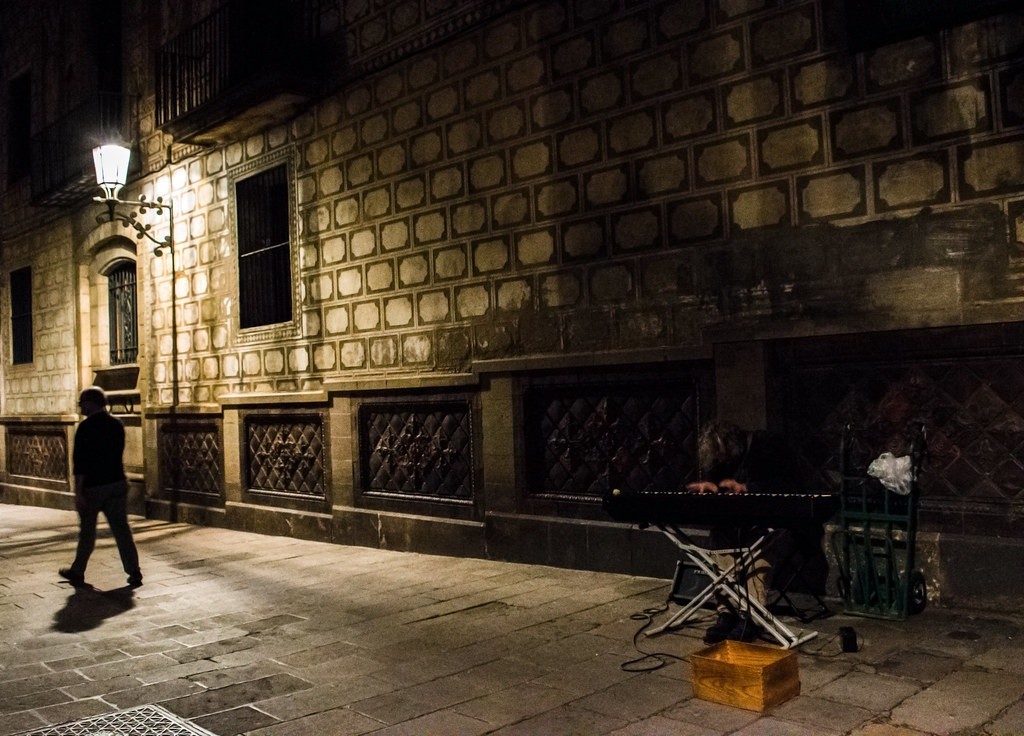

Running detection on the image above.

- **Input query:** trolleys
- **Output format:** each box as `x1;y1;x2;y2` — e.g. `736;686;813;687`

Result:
831;422;926;623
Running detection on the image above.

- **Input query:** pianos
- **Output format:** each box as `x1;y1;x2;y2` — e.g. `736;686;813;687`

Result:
619;488;842;524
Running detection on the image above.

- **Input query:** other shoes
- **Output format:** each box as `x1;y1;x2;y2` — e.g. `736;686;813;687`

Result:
126;573;144;583
705;612;764;644
57;567;84;583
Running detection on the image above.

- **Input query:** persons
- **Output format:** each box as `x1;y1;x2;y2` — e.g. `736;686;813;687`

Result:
686;419;843;645
58;386;144;587
